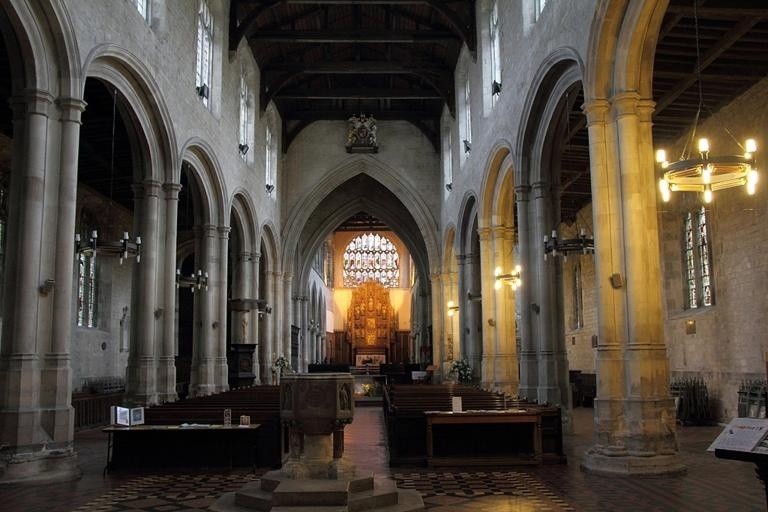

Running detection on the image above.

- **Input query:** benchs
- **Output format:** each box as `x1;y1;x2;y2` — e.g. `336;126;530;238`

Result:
382;380;566;472
99;385;284;477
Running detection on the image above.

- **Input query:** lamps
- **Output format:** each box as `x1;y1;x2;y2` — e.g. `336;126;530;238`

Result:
195;83;276;193
176;164;209;292
654;2;759;204
543;93;594;264
74;88;141;264
445;81;502;192
492;177;522;292
